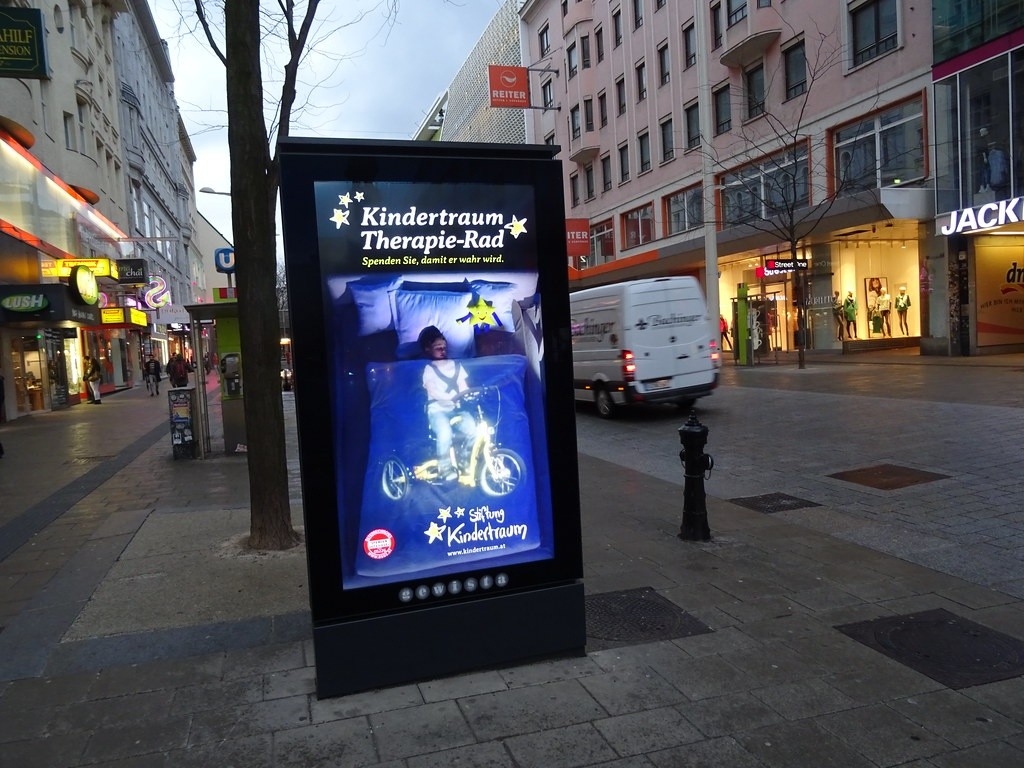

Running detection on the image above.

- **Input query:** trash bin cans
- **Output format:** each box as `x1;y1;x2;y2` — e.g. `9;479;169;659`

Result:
167;386;202;459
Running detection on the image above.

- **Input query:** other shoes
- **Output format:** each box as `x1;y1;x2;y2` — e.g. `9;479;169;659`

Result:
150;392;155;397
87;398;101;405
156;389;159;396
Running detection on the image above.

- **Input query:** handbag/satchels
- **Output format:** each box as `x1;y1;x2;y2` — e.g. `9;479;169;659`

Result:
87;371;100;382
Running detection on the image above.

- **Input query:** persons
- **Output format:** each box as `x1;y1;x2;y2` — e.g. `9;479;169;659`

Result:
82;357;101;404
204;352;211;374
719;314;733;352
895;286;912;336
831;291;845;340
843;289;859;338
978;127;996;194
166;352;194;388
212;353;220;374
422;325;479;487
868;278;882;319
144;354;162;396
875;287;892;338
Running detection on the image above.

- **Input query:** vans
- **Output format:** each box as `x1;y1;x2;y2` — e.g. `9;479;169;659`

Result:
569;276;721;419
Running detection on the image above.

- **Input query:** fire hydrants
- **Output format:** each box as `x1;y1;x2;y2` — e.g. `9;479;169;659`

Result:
677;409;714;541
